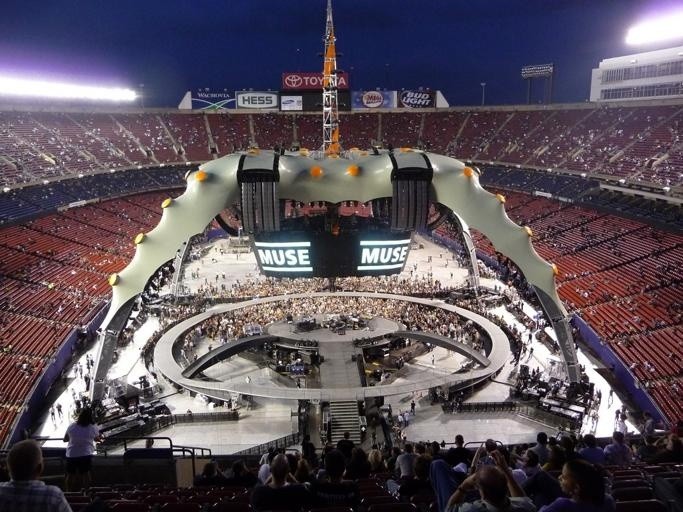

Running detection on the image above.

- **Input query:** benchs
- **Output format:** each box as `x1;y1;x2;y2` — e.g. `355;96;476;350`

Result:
0;109;683;512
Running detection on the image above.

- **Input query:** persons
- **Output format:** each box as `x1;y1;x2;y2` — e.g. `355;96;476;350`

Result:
198;429;682;510
1;439;71;510
338;105;683;395
64;408;100;491
48;235;654;433
2;200;162;432
3;108;324;215
